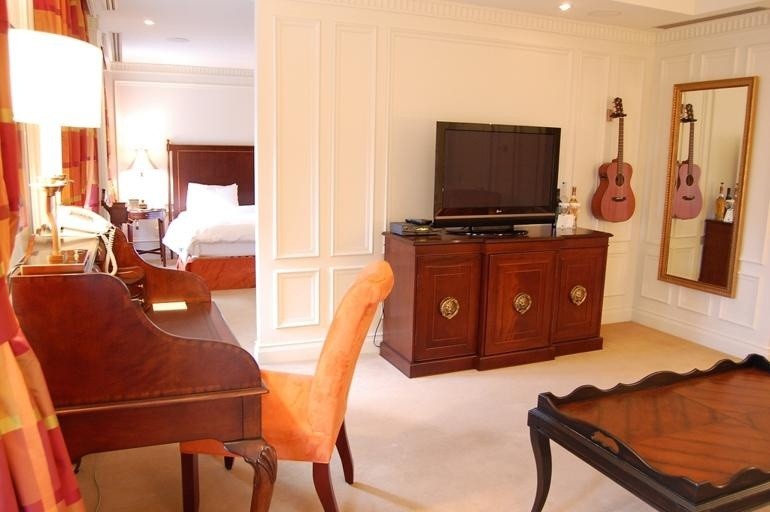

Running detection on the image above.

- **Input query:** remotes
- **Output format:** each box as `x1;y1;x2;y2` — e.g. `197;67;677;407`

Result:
405;219;432;225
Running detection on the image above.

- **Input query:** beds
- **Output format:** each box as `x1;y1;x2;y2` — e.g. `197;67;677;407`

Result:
164;138;255;293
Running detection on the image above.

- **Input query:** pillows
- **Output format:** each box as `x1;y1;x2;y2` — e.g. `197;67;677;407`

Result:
186;181;240;220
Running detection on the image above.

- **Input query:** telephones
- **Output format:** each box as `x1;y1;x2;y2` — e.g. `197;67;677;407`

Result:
54;206;111;236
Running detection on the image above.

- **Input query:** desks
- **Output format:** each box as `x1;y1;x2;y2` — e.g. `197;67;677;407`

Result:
7;224;278;512
127;209;167;267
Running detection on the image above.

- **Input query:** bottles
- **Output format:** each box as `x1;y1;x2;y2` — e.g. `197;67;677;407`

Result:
551;180;579;229
715;181;734;223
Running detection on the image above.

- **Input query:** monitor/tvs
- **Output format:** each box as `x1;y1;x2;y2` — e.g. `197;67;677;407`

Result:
434;121;561;237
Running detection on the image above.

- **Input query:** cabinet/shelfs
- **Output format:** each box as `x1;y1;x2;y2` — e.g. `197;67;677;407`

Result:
379;223;614;377
697;220;733;288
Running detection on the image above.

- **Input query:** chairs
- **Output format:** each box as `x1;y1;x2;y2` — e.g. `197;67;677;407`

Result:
178;261;395;512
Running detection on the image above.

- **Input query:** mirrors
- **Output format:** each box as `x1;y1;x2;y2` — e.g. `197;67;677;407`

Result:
657;76;761;299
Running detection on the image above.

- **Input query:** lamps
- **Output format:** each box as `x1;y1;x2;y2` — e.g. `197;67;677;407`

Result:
6;25;105;275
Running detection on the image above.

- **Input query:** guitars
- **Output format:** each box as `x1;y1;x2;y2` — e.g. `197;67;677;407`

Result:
674;103;702;218
592;97;635;222
